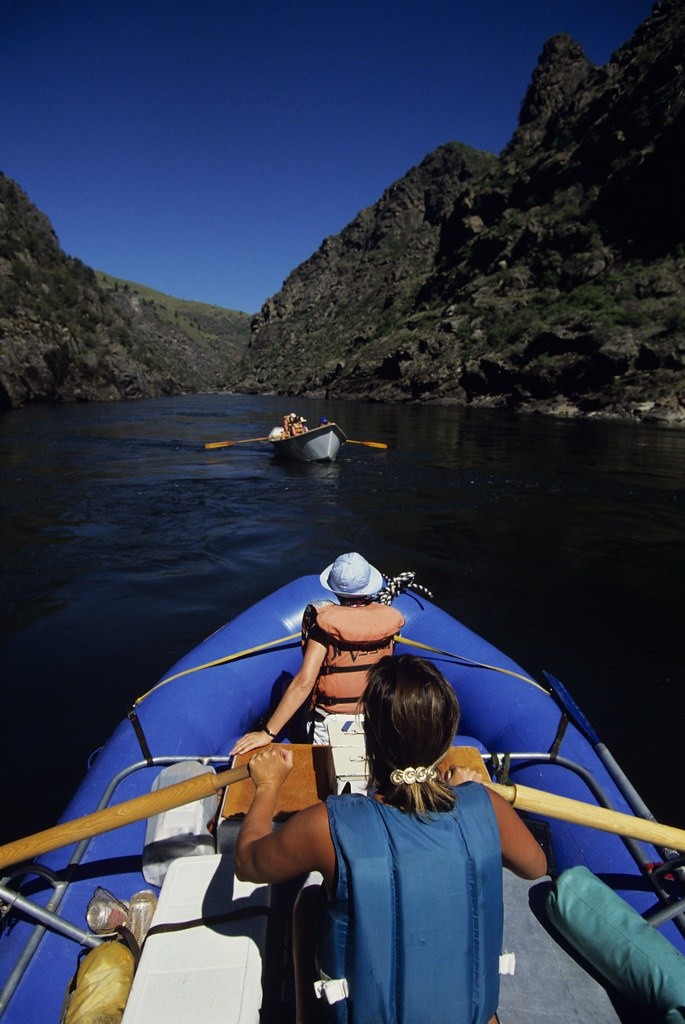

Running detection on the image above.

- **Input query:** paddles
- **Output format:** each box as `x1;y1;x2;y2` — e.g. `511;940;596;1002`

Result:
346;439;387;448
1;763;251;870
480;779;685;851
206;432;284;450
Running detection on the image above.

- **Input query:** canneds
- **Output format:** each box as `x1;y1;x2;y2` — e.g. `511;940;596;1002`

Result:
127;889;157;947
87;899;130;934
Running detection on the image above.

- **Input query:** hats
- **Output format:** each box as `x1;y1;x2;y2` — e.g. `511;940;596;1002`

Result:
290;413;296;421
320;552;383;598
320;417;327;422
300;417;307;422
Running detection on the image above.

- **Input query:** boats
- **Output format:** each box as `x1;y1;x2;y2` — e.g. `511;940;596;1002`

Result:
269;420;348;464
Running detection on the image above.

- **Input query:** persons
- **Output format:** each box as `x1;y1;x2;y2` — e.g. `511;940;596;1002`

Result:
284;413;331;439
232;653;547;1024
230;553;405;754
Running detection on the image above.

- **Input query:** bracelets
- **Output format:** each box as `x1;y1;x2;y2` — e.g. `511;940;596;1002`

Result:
263;725;277;738
